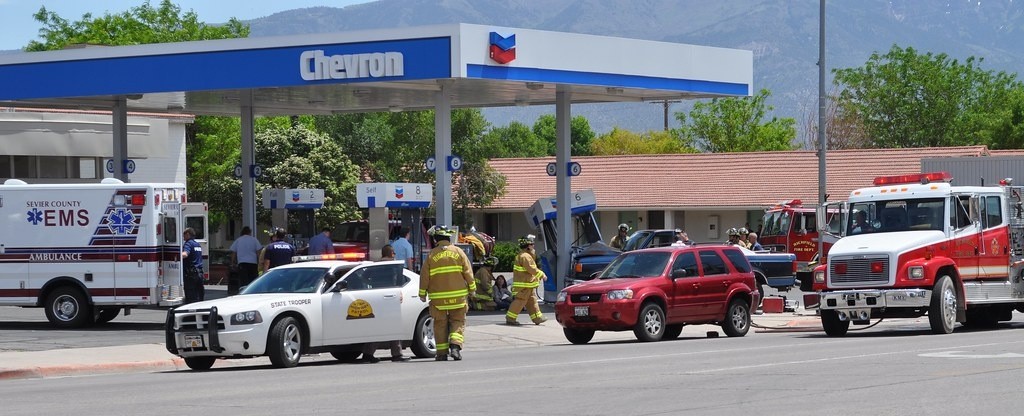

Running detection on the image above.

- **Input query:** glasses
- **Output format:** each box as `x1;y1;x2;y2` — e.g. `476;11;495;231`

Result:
393;253;396;255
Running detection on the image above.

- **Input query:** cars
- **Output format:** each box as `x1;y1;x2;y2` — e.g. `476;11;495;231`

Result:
164;253;436;372
208;250;234;283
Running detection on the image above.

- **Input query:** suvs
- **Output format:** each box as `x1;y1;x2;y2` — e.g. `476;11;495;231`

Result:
555;246;760;344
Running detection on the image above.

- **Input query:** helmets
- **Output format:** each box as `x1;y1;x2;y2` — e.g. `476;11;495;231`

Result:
482;258;494;267
517;234;536;248
617;223;630;237
726;228;740;244
737;227;749;235
433;224;456;242
268;226;280;242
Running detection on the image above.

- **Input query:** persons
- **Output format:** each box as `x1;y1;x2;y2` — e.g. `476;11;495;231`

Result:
724;227;763;251
359;245;412;364
418;225;477;360
467;257;527;311
608;223;635;251
390;227;414;272
308;227;335;254
182;227;205;305
504;234;548;325
229;226;298;293
679;231;697;245
853;210;873;233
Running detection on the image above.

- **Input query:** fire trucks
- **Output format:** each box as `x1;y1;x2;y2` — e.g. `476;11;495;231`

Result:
803;172;1024;337
758;200;861;290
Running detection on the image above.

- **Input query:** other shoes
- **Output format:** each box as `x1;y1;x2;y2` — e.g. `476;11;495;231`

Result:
434;352;448;361
361;354;378;362
534;316;548;325
469;303;474;310
393;356;412;361
451;348;463;360
506;321;519;325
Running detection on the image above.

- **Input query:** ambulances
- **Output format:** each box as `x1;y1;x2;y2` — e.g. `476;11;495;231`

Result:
0;178;211;328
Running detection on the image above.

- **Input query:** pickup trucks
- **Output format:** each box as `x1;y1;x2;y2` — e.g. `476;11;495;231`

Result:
564;230;802;303
297;213;474;280
